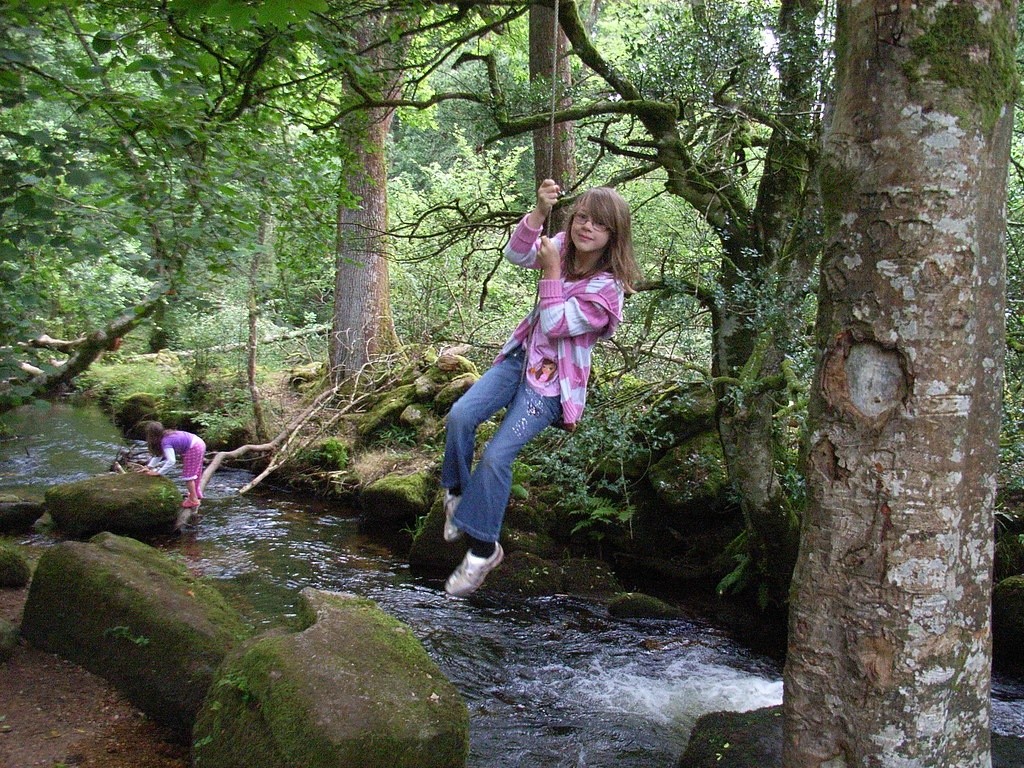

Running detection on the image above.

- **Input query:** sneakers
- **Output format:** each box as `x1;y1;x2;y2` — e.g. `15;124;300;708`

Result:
445;541;504;596
444;489;465;541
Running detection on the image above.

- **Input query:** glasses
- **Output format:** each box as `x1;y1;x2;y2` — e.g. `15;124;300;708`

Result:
575;212;613;235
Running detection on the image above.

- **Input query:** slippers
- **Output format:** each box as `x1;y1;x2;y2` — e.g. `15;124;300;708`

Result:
186;492;191;498
182;498;199;507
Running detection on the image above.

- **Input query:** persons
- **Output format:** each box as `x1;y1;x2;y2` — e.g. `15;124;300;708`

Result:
438;180;637;594
137;420;206;508
